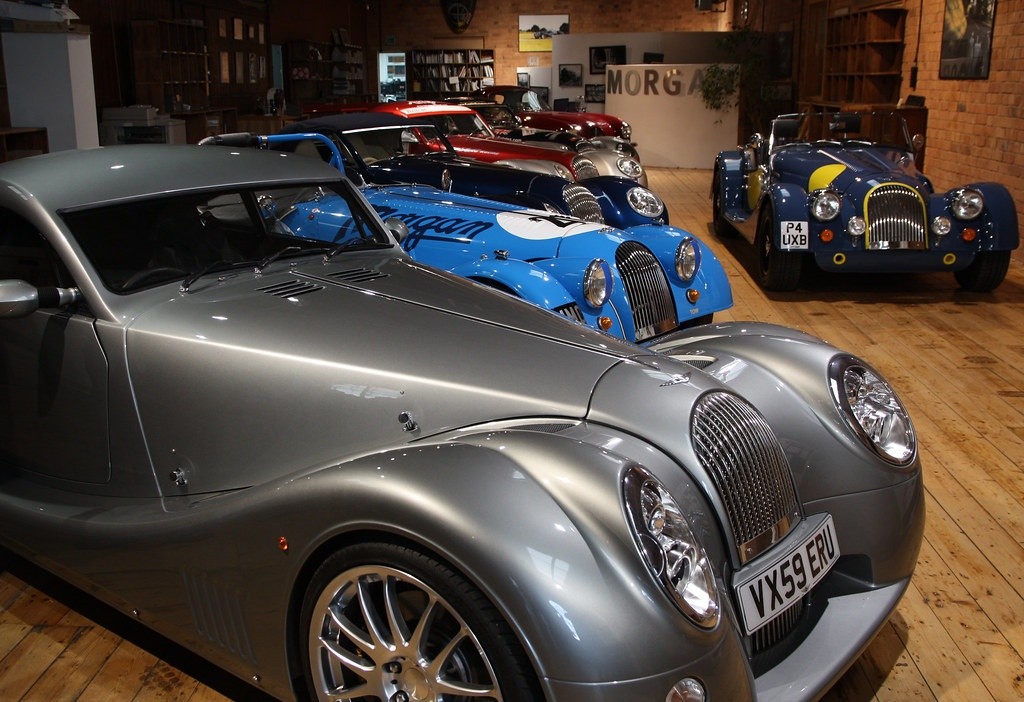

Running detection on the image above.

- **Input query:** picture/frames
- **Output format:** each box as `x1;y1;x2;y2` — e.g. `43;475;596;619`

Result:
936;0;997;81
517;12;569;52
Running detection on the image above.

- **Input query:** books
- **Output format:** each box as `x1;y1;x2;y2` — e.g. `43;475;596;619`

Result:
412;50;494;92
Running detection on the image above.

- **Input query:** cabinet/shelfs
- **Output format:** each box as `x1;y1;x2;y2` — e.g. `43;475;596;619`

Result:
793;0;927;177
284;38;364;108
588;43;626;73
584;83;604;102
131;15;239;145
411;46;496;101
0;125;49;164
558;62;582;87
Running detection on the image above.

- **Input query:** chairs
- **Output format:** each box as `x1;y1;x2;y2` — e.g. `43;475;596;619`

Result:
837;111;858;142
772;118;798;146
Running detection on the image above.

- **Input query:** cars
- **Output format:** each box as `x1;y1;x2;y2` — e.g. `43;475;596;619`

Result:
0;143;927;702
716;109;1020;300
201;82;732;343
